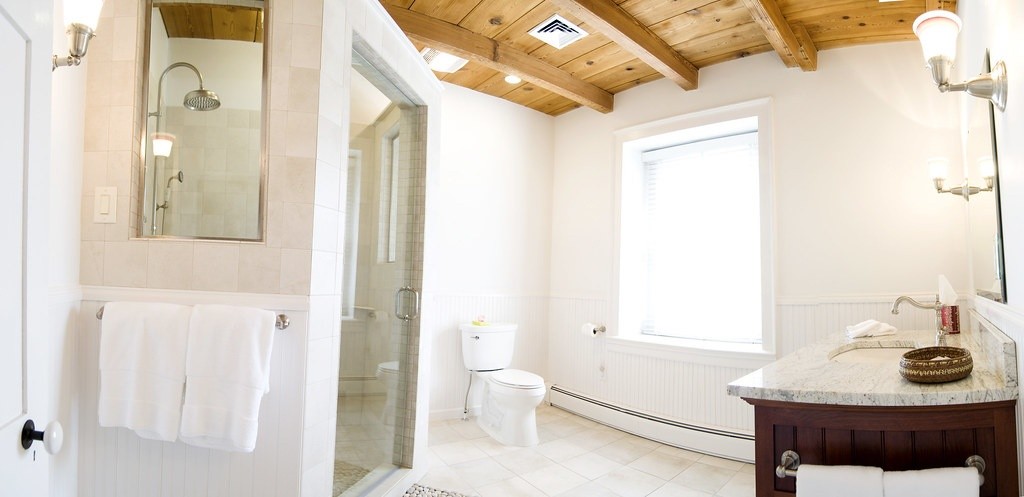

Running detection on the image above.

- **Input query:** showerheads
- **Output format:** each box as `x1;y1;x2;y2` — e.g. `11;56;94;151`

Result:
183;89;221;112
172;170;184;183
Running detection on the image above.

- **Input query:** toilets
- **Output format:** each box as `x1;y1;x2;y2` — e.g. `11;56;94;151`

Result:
452;316;547;447
376;359;400;426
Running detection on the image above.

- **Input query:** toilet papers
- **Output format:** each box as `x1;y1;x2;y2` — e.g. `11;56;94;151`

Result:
581;322;599;338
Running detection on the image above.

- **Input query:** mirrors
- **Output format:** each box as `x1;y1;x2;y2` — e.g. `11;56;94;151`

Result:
965;50;1005;304
135;0;273;245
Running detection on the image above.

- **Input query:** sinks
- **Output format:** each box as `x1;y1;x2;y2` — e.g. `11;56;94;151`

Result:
828;340;926;365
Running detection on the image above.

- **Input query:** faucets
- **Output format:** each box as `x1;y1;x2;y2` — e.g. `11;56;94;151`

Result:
891;295;945;315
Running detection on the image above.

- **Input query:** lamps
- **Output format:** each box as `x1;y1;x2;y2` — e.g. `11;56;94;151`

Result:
50;0;102;68
913;10;1007;111
921;120;993;200
150;130;176;157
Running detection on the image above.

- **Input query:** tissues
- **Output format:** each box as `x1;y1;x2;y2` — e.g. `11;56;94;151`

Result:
937;273;961;335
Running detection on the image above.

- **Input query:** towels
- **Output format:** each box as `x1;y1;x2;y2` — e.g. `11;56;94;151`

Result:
844;319;898;339
795;463;980;497
97;302;276;453
